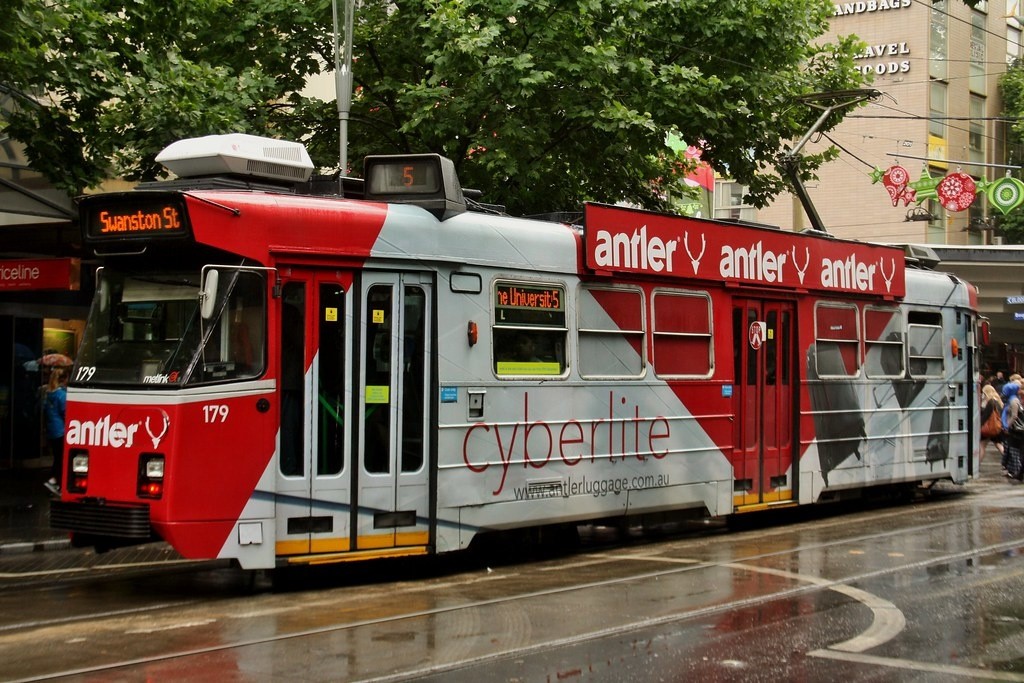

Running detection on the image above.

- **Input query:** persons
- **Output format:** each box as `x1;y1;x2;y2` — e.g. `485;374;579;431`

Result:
42;366;69;496
1000;383;1024;480
979;372;1024;407
978;385;1005;464
512;331;545;362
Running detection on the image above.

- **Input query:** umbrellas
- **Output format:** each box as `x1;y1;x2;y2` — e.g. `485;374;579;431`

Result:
23;361;38;372
36;354;74;366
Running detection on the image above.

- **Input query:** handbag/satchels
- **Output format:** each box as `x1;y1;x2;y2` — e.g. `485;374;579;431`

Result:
981;410;1002;437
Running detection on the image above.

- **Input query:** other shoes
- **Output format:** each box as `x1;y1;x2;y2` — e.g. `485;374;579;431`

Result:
45;481;61;495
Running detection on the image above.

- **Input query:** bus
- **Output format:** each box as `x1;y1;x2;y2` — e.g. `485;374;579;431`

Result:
47;88;990;596
